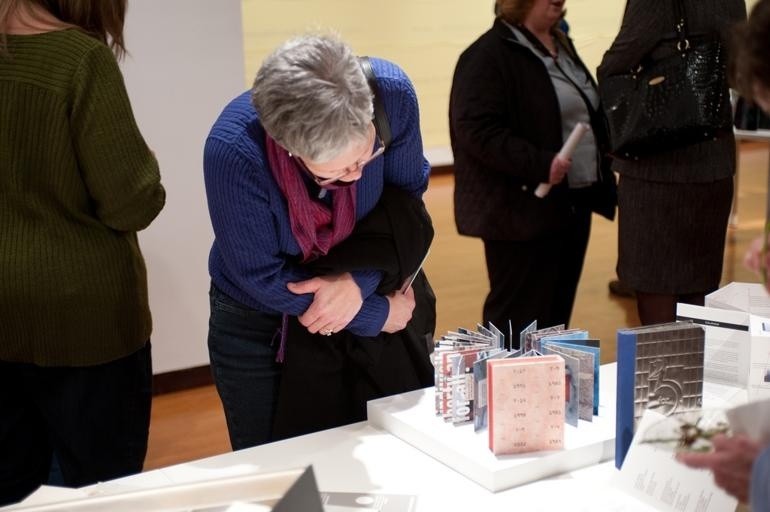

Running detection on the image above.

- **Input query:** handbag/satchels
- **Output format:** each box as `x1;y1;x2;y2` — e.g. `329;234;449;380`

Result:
595;38;726;167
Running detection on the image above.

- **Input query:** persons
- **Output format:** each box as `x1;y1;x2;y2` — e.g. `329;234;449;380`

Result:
2;2;166;508
598;1;749;324
673;0;770;512
202;29;432;453
447;1;617;351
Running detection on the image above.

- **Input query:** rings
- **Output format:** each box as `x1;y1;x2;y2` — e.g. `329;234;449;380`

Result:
321;327;333;337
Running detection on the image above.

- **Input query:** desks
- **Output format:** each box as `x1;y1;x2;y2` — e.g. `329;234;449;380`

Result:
3;329;766;509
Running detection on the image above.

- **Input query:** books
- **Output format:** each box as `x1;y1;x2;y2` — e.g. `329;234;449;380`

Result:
485;355;565;455
614;321;705;472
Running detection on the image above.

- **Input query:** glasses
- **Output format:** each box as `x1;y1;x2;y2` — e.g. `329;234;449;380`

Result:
289;134;387;188
